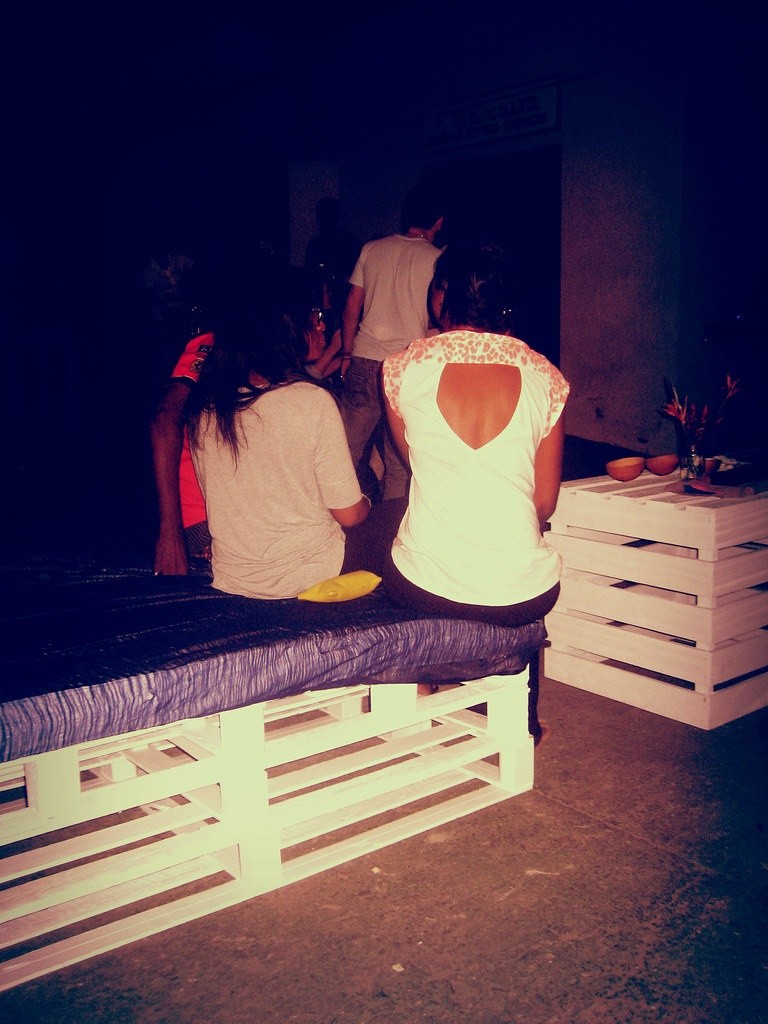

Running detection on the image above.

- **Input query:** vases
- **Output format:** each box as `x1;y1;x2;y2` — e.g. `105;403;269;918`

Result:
677;436;712;482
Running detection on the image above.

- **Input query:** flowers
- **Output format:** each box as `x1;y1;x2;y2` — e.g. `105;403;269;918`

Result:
662;374;742;476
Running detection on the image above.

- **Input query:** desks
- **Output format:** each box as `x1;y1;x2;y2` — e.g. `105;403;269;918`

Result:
540;475;768;732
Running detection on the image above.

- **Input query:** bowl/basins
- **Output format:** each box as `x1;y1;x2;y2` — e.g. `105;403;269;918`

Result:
606;457;644;481
645;454;678;475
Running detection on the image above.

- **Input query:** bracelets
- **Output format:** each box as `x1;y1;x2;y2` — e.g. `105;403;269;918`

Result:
343;352;351;360
362;494;372;509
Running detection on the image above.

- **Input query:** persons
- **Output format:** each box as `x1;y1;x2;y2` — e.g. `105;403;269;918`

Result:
150;190;451;601
379;278;570;743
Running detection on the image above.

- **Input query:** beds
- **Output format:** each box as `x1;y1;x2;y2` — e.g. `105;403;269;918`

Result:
1;563;533;997
